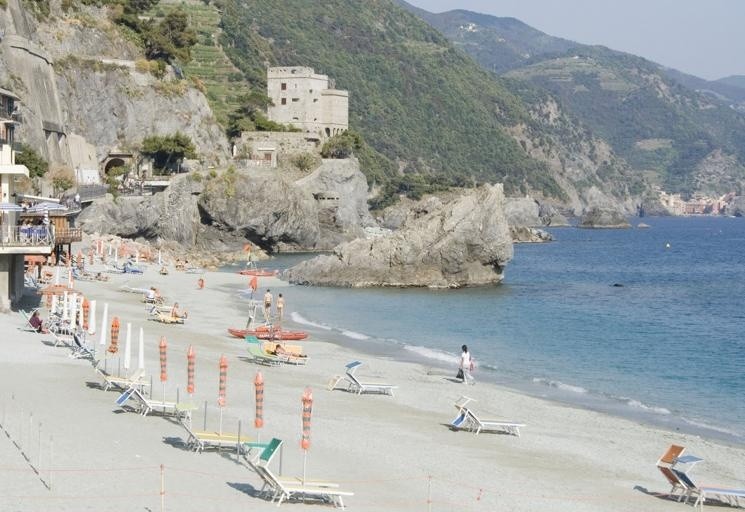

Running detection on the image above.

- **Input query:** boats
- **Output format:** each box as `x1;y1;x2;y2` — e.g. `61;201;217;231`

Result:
227;323;310;341
238;270;275;276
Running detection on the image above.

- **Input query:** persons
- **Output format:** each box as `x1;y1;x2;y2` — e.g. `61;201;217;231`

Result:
264;288;272;318
154;288;166;303
16;219;56;244
199;276;204;288
249;276;257;291
147;287;156;301
274;343;307;358
275;293;285;320
459;344;476;385
29;310;45;333
172;301;187;319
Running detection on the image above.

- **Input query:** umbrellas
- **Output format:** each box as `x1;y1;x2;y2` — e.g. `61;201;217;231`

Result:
25;236;163;289
252;367;265;463
107;315;120;376
43;288;108;373
158;335;169;404
0;202;24;246
185;343;197;431
123;322;131;381
217;352;228;435
137;326;147;395
26;200;69;244
299;385;314;485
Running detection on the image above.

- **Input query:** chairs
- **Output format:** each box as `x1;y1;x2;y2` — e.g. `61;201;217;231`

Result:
74;251;192;308
328;360;398;398
439;394;528;438
92;361;146;394
243;437;355;511
18;307;97;361
655;444;745;512
243;335;311;368
172;404;256;458
145;308;189;325
115;385;178;417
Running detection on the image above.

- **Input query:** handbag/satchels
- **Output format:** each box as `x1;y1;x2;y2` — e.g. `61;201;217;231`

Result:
455;369;464;379
